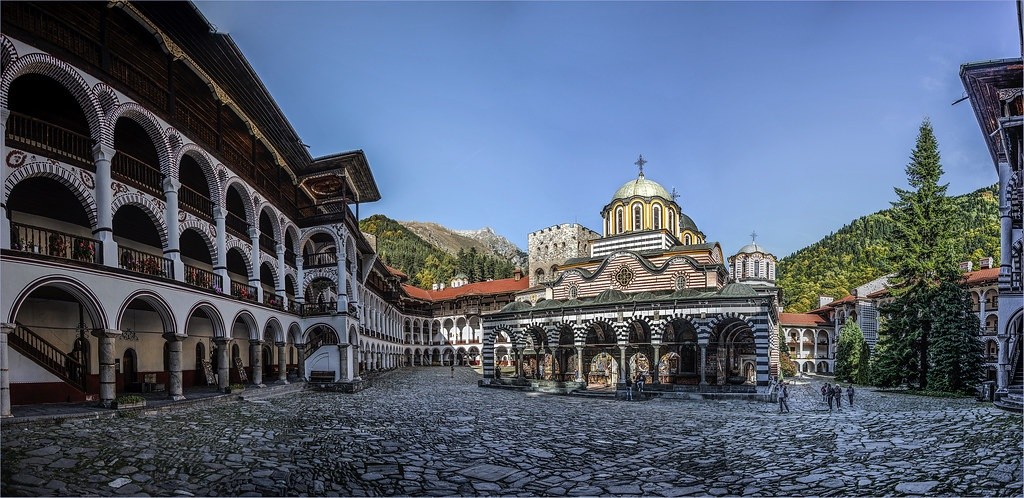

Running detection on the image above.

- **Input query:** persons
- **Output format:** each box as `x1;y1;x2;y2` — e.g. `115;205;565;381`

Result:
637;372;646;391
574;369;578;379
834;384;842;410
847;384;854;405
625;376;633;400
779;386;790;413
495;362;501;378
821;382;833;413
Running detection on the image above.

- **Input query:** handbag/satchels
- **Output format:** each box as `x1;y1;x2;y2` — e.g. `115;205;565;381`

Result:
783;387;789;398
